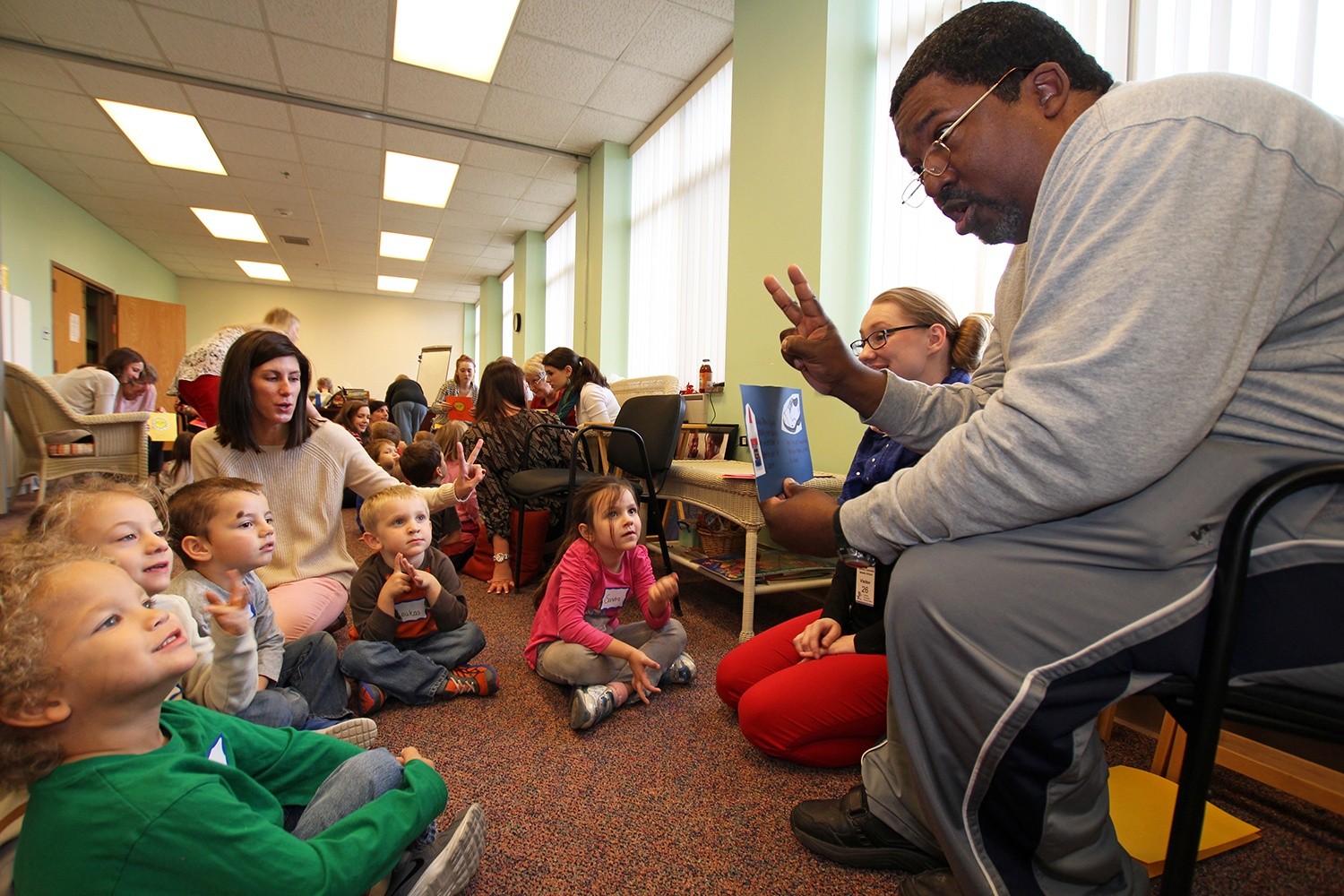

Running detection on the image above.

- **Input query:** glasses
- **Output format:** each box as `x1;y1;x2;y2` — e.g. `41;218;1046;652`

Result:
900;66;1036;208
850;325;930;357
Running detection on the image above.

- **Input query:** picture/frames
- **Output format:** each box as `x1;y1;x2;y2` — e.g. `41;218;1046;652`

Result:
672;420;740;460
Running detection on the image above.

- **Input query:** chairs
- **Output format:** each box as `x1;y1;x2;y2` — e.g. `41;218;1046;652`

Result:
1;362;153;513
507;393;689;619
1141;452;1342;896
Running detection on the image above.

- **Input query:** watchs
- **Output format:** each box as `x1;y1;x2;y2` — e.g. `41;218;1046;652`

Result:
833;504;877;570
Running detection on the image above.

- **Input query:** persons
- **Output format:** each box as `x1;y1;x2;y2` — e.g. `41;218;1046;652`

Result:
1;302;702;895
713;287;990;767
757;1;1343;896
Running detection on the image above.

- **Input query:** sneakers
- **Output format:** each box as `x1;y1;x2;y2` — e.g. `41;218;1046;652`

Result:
898;868;965;896
298;716;378;750
570;684;615;731
789;782;940;873
447;662;499;700
381;802;487;896
659;651;698;684
357;680;387;716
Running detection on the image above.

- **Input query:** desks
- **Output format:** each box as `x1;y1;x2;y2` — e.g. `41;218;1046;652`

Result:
638;457;853;647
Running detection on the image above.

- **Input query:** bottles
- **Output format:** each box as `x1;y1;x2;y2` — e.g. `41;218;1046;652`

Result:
698;358;713;393
